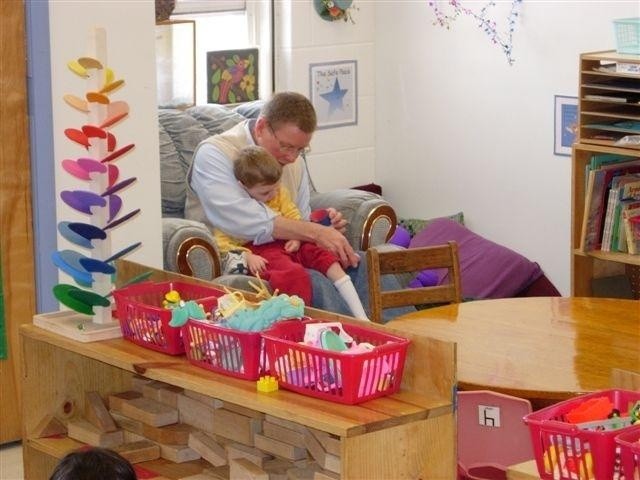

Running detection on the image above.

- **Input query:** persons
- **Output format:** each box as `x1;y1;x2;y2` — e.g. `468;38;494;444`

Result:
184;90;418;326
212;145;368;321
49;446;139;480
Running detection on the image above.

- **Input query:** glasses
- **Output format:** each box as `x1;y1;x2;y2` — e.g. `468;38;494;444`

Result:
267;121;311;154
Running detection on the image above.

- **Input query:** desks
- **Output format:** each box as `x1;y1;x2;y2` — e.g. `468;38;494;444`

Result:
384;297;640;409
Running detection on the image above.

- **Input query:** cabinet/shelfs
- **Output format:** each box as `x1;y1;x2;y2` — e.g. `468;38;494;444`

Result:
570;49;640;300
18;259;456;480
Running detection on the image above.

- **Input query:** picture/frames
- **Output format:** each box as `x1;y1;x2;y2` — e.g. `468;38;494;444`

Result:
309;60;358;130
554;95;579;157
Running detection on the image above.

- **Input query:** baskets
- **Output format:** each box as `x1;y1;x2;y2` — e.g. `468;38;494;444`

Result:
112;281;225;356
523;388;640;480
181;311;311;381
613;17;640;55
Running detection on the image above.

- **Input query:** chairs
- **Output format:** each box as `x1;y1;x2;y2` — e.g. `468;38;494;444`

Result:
455;390;533;480
367;239;462;325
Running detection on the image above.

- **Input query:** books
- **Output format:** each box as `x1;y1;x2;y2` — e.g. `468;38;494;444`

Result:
579;154;640;256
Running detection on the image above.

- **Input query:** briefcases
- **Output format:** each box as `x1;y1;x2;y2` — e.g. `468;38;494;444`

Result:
260;320;410;405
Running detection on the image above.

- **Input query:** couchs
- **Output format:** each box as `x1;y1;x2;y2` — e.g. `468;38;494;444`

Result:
158;100;397;282
